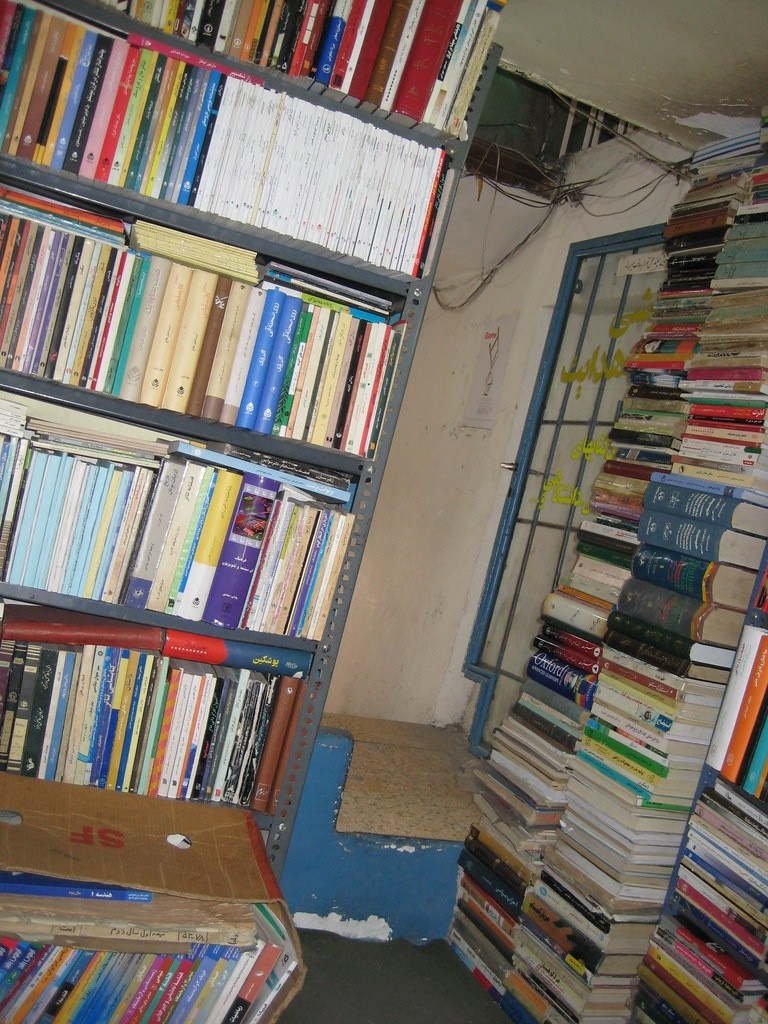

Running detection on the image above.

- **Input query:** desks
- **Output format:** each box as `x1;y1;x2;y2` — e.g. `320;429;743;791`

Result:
0;0;504;890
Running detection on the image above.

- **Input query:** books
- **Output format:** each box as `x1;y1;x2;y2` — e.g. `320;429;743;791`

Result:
445;126;767;1024
1;812;299;1023
0;0;510;812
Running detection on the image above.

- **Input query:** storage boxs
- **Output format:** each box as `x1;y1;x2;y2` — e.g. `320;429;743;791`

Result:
0;766;309;1024
